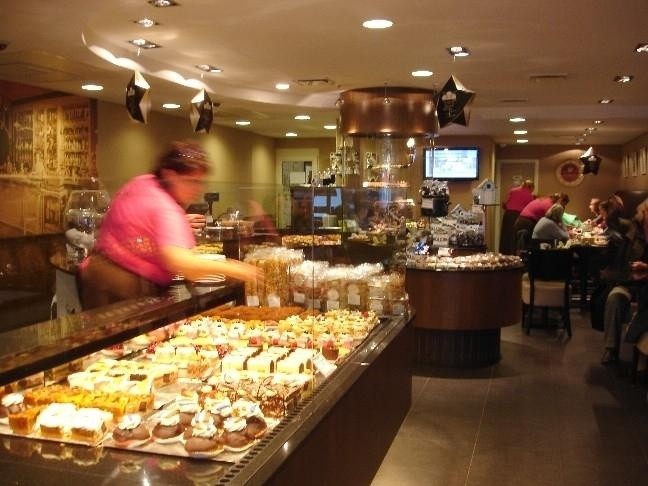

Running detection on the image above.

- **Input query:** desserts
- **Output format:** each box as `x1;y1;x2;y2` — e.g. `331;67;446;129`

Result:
0;304;383;459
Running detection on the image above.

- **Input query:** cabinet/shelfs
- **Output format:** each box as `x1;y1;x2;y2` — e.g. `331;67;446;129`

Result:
0;97;97;235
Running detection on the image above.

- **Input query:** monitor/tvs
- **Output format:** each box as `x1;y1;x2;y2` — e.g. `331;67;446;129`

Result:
186;203;209;214
423;147;479;181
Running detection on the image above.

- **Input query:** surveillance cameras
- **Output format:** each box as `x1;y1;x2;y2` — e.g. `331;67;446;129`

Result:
0;40;10;51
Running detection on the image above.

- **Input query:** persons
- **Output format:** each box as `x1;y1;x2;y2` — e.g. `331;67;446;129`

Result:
499;181;648;364
76;140;264;308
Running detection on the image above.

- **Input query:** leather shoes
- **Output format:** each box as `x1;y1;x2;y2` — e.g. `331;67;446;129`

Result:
601;348;619;363
